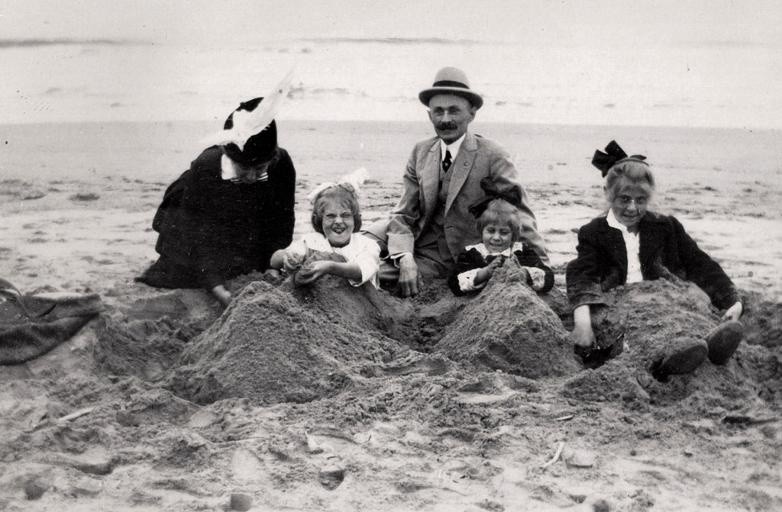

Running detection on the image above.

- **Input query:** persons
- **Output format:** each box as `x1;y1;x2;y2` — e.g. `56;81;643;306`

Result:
445;199;555;297
130;81;298;309
269;173;380;294
563;138;744;376
365;65;551;300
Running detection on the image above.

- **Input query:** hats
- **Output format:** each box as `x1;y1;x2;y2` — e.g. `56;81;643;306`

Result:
224;97;277;166
418;68;483;109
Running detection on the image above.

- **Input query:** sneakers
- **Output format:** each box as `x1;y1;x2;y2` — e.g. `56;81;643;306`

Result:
702;320;743;365
655;340;708;374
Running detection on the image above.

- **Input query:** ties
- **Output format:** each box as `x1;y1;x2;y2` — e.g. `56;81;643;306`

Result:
442;150;454;173
